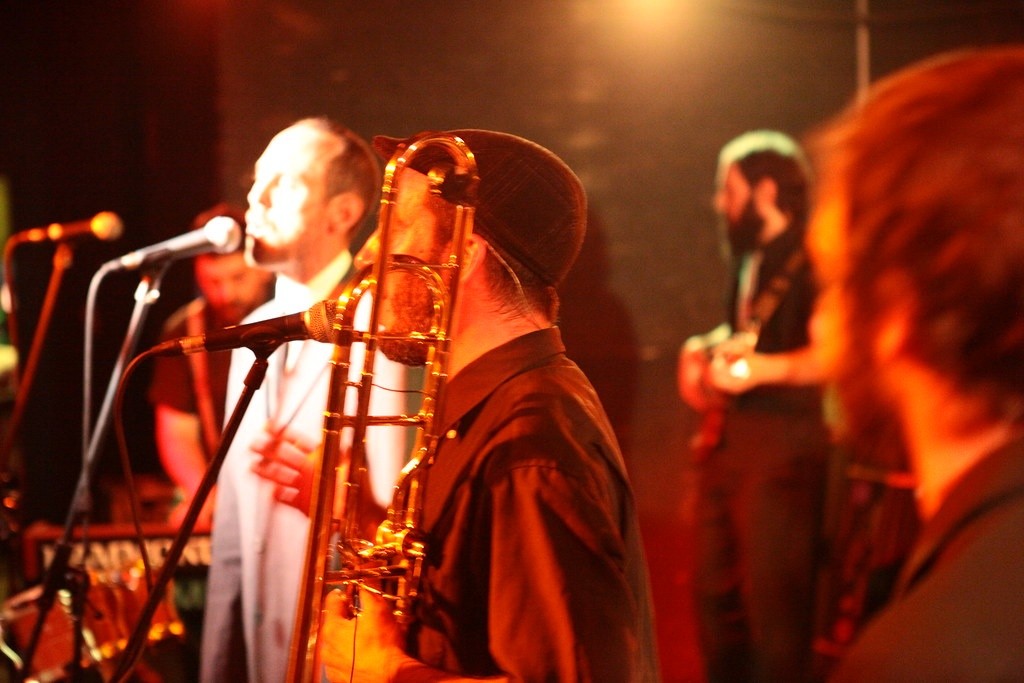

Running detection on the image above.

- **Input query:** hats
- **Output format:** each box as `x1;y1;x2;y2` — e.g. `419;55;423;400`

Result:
374;128;585;286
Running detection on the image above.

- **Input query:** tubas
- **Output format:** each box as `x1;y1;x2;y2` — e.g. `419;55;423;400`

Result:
283;131;479;683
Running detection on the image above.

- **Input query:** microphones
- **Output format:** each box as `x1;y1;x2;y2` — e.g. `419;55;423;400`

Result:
154;299;342;358
18;213;125;242
105;216;242;271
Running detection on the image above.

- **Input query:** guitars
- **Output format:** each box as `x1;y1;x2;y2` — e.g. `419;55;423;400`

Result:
685;326;762;453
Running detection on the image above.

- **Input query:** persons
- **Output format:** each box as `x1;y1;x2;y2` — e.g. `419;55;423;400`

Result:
678;130;848;682
249;130;664;682
199;114;427;682
802;41;1024;682
153;203;274;526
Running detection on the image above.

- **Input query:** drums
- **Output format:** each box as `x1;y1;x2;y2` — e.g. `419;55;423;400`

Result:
1;562;184;683
